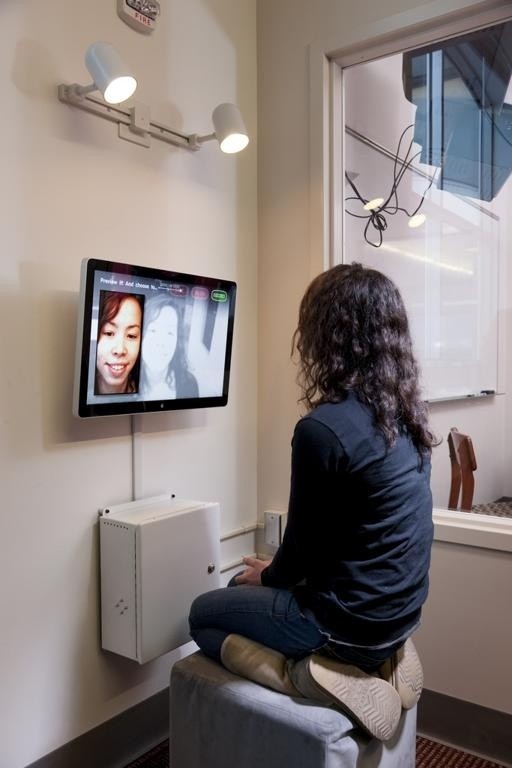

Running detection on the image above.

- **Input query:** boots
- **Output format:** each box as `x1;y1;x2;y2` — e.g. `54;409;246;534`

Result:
219;631;403;741
378;635;425;710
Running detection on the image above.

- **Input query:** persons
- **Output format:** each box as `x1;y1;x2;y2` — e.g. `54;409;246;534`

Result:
94;289;143;394
106;292;200;402
188;261;444;743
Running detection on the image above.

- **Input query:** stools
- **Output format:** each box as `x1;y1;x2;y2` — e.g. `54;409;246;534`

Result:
165;643;421;767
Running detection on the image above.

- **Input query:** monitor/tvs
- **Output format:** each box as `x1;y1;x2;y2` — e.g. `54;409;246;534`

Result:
72;256;238;419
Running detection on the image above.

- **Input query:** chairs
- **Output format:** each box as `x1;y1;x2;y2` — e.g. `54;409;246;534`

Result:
445;424;512;518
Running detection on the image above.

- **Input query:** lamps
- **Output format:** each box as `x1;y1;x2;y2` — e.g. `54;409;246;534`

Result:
58;40;250;157
